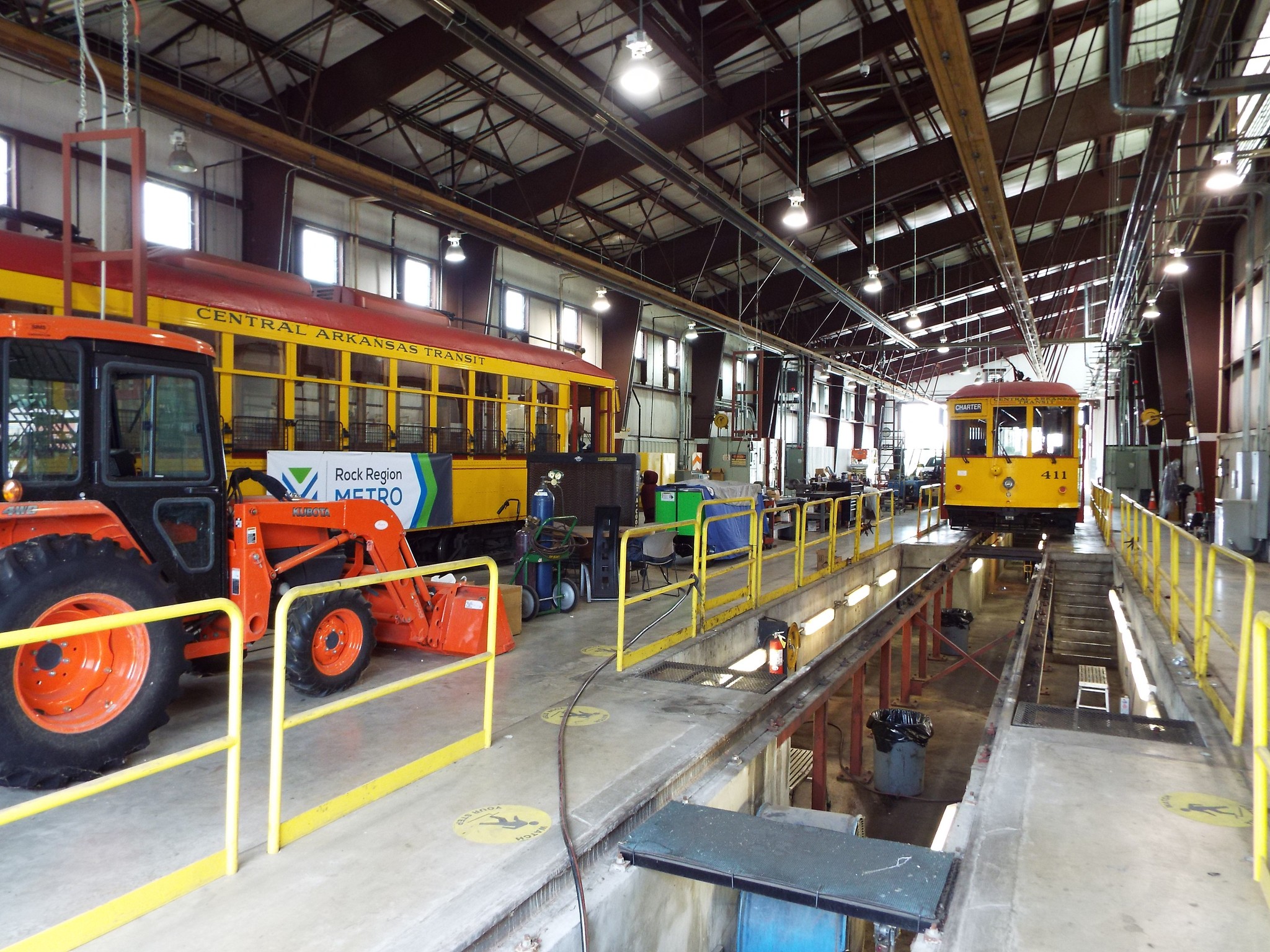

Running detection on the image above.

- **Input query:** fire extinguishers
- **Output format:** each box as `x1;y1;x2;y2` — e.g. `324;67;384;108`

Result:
761;630;787;674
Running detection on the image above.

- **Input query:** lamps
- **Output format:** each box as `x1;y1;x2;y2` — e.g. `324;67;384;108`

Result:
1097;0;1241;393
166;40;198;173
862;130;883;295
685;204;997;396
1108;583;1170;731
445;128;466;262
700;568;898;688
592;245;611;312
620;0;659;94
782;0;808;228
969;558;984;573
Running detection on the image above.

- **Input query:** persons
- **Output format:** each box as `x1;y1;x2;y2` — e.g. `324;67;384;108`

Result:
569;421;586;452
641;470;658;523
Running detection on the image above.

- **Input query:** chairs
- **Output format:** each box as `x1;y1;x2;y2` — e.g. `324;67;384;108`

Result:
522;523;680;601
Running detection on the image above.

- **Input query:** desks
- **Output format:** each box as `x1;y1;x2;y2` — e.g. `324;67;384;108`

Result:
796;491;845;533
767;499;798;546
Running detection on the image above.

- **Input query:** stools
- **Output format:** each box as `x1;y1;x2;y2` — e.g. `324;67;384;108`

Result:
1073;665;1110;731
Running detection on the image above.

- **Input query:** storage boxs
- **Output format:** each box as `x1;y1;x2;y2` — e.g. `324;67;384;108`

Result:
710;468;725;482
816;548;842;571
808;521;820;532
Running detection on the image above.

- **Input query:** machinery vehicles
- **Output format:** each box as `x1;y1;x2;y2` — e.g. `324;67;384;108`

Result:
0;298;519;792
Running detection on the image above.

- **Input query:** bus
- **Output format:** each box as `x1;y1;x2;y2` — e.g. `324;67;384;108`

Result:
0;200;633;626
945;378;1089;539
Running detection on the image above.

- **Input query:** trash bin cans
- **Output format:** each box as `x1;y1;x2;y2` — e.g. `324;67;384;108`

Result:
1213;497;1252;551
939;607;974;656
865;709;935;798
